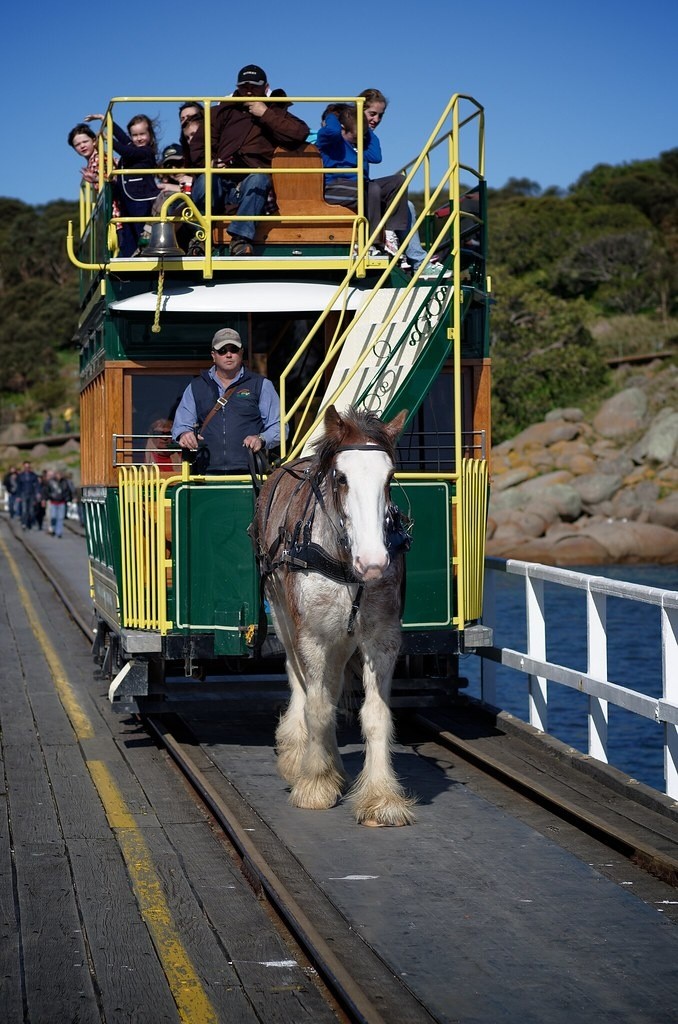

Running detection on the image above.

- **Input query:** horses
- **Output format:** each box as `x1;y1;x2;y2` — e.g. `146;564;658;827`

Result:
254;404;424;829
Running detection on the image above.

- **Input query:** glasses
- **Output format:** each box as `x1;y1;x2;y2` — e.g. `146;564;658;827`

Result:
163;160;184;169
151;431;172;436
214;347;240;355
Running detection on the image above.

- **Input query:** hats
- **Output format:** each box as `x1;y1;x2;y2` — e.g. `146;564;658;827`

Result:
161;144;185;163
236;64;267;87
211;328;242;350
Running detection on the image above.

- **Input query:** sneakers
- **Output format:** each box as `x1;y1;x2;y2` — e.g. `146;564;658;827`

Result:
229;237;254;255
400;253;440;268
383;235;403;259
351;242;382;257
413;261;452;280
185;237;215;256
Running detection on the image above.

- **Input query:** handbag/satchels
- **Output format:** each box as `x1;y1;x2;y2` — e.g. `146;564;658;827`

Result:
180;385;239;462
181;433;200;462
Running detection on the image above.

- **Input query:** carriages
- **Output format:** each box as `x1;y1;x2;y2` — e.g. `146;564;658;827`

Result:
66;94;494;830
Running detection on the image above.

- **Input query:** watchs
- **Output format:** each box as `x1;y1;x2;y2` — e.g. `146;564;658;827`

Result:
256;433;266;449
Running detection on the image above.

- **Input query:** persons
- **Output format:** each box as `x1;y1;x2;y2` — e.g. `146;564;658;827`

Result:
179;101;203;125
170;327;289;475
41;403;76;436
83;113;158;258
191;64;310;256
3;460;76;539
313;102;409;259
159;143;187;184
138;115;205;244
131;408;182;472
350;89;454;280
68;122;128;258
